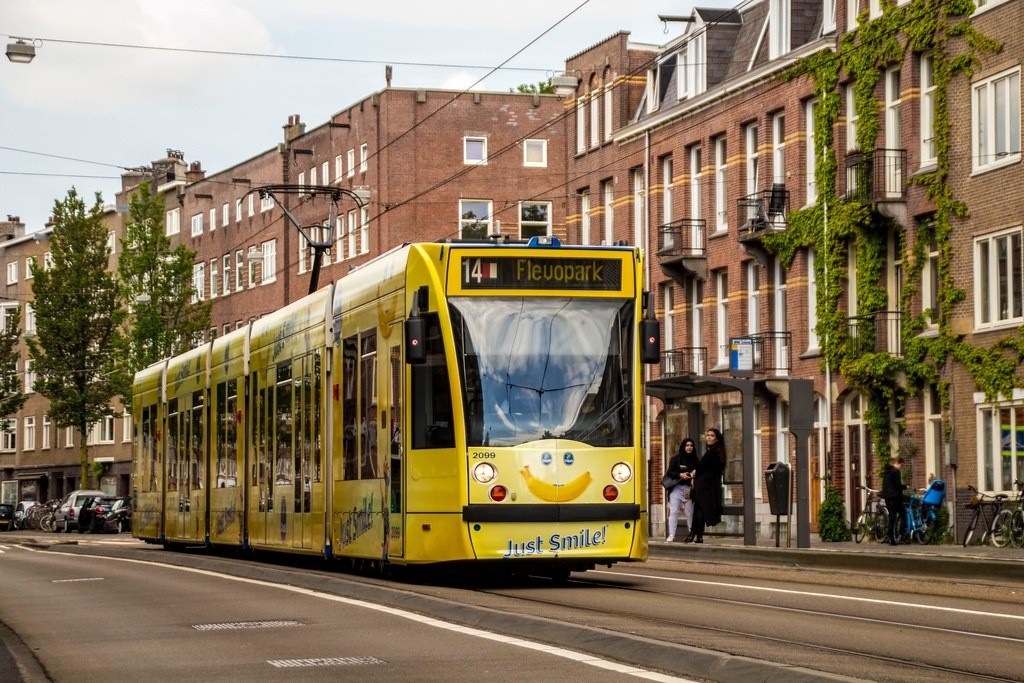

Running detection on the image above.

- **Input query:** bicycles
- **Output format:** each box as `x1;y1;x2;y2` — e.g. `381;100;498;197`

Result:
854;484;890;544
23;498;62;532
962;484;1015;548
893;485;942;545
1009;479;1024;549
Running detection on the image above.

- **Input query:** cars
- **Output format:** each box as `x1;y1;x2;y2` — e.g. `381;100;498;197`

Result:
78;496;116;533
13;501;41;529
105;496;133;533
0;504;15;529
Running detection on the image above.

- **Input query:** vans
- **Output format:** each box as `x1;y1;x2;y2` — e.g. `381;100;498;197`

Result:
51;490;108;533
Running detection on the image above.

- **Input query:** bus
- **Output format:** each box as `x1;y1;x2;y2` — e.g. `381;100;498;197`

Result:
133;183;649;575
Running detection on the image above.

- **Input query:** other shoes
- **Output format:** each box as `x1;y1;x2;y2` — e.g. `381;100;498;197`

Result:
898;535;904;542
889;538;897;544
685;535;694;542
666;537;676;542
695;536;704;542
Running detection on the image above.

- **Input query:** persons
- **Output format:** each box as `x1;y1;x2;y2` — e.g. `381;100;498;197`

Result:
878;457;912;545
666;437;699;543
684;428;727;543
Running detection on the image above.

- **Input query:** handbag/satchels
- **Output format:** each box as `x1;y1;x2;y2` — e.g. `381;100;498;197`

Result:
661;470;683;490
